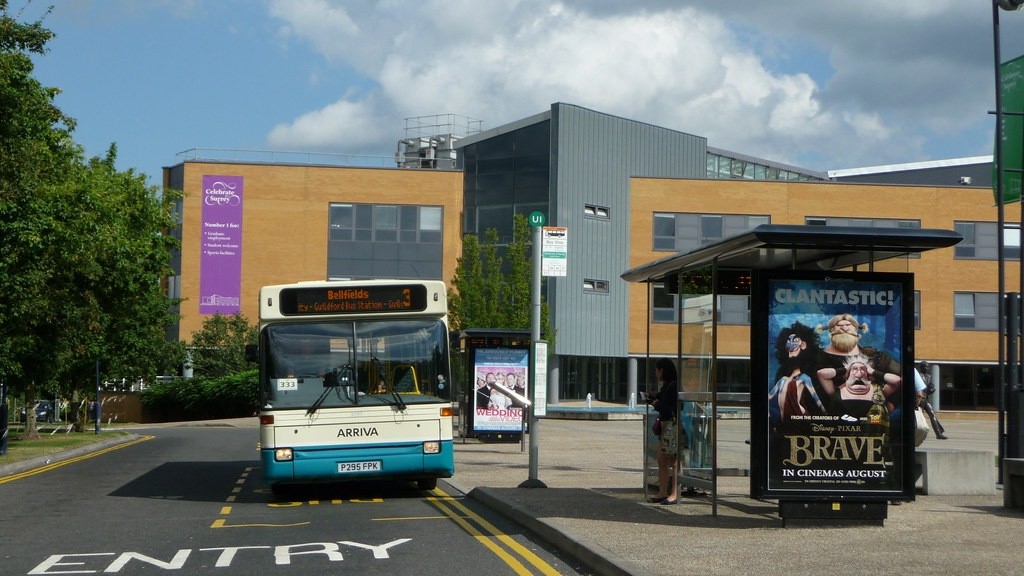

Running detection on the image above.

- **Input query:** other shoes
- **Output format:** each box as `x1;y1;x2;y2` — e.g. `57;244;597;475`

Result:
647;496;668;503
661;498;677;505
937;434;948;439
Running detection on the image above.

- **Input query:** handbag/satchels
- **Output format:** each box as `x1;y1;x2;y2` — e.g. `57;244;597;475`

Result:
653;417;662;435
913;406;929;447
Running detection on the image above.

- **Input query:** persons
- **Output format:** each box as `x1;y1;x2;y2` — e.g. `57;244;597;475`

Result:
298;341;326;381
477;373;524;409
88;399;95;419
919;361;948;439
644;358;684;505
915;361;927;406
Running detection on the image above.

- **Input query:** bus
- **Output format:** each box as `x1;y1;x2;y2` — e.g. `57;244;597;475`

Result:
243;277;461;494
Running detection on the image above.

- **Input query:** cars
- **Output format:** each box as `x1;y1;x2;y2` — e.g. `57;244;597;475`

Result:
20;399;55;424
